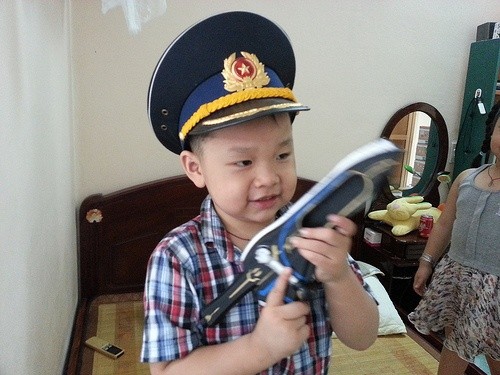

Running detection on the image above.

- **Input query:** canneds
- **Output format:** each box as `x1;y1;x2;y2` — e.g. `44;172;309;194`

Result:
417;214;433;238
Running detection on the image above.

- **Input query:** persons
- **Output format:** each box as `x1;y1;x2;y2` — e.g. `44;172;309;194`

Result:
140;13;379;375
408;103;500;374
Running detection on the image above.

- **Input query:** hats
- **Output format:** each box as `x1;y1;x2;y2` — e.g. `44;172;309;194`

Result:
147;11;311;156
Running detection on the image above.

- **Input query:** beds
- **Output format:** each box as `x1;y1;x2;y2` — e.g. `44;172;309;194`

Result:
63;172;488;375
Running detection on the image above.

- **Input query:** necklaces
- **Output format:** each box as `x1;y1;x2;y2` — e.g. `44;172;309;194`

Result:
488;167;500;187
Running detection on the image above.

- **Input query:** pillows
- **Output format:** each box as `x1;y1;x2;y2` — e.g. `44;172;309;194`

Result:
362;261;407;336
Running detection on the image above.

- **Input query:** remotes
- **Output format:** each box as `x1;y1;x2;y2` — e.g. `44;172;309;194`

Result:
85;336;124;359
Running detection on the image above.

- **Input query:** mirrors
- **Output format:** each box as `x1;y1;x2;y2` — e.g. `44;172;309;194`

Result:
387;111;439;199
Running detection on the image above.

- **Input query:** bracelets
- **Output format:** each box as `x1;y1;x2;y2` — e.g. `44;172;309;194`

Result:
419;252;437;267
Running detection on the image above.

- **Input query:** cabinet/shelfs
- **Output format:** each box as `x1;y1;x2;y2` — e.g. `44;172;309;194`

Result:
453;38;500;194
366;220;451;323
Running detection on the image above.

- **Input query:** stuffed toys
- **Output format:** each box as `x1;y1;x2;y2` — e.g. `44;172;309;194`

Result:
369;196;442;236
